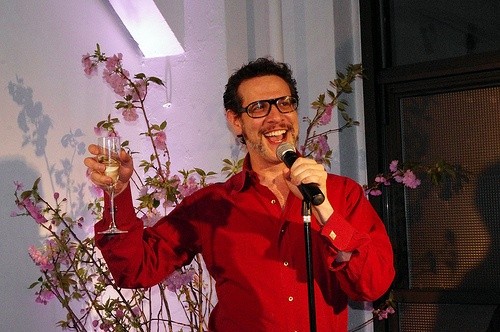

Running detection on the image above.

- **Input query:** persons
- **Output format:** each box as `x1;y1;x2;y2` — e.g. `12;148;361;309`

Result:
84;58;396;332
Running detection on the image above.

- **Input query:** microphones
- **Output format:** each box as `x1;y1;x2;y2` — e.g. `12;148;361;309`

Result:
276;141;325;206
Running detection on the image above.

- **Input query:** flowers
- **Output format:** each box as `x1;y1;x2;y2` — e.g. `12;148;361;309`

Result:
10;44;469;332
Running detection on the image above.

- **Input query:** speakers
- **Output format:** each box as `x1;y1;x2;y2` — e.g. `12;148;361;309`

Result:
370;0;500;86
386;299;500;332
379;70;500;299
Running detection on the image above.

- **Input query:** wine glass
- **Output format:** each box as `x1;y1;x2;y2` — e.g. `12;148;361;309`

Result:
95;136;129;234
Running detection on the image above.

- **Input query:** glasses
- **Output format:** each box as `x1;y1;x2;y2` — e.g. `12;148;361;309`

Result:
237;96;298;118
